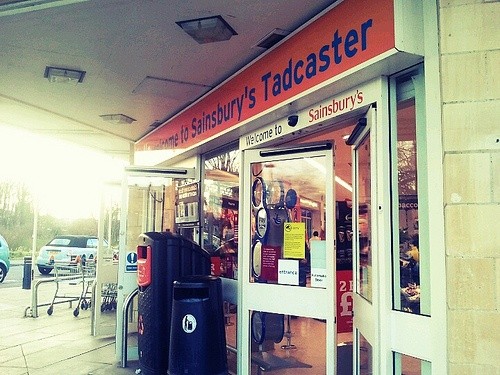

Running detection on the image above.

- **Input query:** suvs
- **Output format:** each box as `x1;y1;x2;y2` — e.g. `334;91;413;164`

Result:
35;233;118;274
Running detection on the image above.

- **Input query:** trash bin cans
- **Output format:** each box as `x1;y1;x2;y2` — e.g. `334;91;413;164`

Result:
168;280;226;372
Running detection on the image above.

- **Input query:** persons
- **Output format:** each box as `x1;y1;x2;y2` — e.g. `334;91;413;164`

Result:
290;226;419;319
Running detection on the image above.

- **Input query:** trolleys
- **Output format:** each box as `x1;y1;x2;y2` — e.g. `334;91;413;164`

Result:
47;259;119;318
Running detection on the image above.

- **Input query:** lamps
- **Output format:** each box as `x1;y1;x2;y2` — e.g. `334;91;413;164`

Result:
43;66;86;87
175;15;238;45
100;114;137;128
